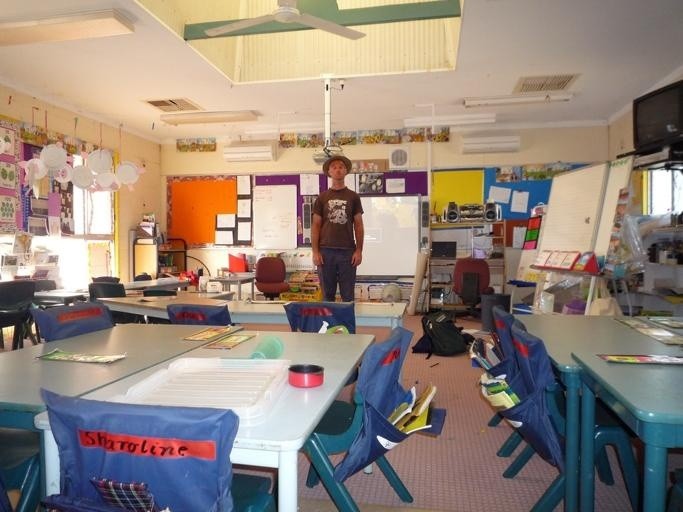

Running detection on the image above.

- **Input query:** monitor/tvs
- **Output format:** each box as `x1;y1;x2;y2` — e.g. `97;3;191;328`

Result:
632;81;683;149
431;241;457;259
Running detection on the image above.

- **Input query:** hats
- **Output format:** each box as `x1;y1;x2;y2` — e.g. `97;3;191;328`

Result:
323;154;352;176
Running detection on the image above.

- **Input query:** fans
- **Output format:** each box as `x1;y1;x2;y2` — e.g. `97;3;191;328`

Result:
205;0;368;42
388;145;409;171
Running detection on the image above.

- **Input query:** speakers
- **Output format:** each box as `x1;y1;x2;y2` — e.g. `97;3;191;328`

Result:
389;144;408;169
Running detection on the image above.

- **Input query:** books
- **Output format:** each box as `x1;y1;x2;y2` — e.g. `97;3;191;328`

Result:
386;380;438;436
470;330;503;370
479;372;520;411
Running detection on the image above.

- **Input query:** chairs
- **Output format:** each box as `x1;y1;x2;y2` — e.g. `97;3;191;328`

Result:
283;301;357;334
36;387;240;512
23;279;56;338
452;259;493;319
1;278;38;350
26;301;115;342
89;281;139;324
487;303;569;459
217;266;238;293
497;320;643;512
166;303;232;327
145;287;178;324
92;275;120;288
131;273;151;281
300;327;449;503
254;257;290;301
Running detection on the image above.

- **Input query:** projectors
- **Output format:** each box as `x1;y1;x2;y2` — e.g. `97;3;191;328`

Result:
313;146;345;164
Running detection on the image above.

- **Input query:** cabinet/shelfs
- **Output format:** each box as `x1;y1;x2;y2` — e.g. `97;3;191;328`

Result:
426;220;506;314
640;224;683;234
642;259;682;268
132;237;187;280
615;127;682;170
633;284;681;297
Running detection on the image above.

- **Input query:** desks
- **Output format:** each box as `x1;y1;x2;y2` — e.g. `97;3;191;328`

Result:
571;350;682;510
33;329;376;510
0;322;246;510
98;295;231;324
217;300;407;329
615;315;683;356
209;276;257;302
124;277;188;296
176;291;234;300
30;290;87;342
638;316;682;337
510;311;683;510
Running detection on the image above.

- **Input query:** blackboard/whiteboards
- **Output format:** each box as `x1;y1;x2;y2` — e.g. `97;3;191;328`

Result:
532;155;635;264
355;194;420;277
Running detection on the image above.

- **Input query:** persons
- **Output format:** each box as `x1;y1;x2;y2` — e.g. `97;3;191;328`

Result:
311;155;364;302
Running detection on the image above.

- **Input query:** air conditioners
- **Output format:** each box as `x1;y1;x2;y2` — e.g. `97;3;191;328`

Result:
220;139;281;163
454;130;522;153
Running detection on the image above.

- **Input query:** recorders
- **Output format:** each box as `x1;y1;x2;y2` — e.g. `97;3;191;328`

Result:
448;202;501;222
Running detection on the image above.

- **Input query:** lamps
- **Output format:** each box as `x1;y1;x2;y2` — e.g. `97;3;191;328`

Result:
462;92;571;107
404;113;498;128
0;9;136;47
160;110;259;125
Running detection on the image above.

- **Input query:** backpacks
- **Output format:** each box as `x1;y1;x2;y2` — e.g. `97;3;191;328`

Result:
421;310;466;360
